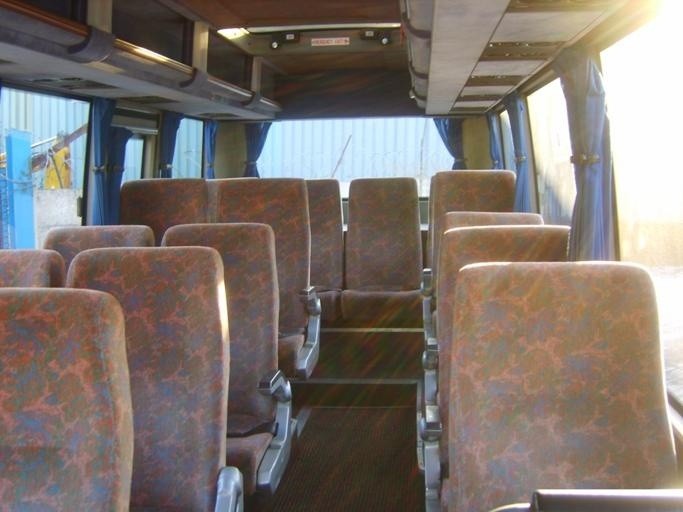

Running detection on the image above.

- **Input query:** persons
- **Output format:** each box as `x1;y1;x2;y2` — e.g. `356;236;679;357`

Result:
43;129;70;190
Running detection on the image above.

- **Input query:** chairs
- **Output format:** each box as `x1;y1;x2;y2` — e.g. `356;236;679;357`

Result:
0;284;138;512
411;169;543;470
444;257;682;509
118;176;323;388
304;175;427;320
39;223;154;270
66;246;247;511
157;222;296;498
419;221;575;512
1;245;66;289
